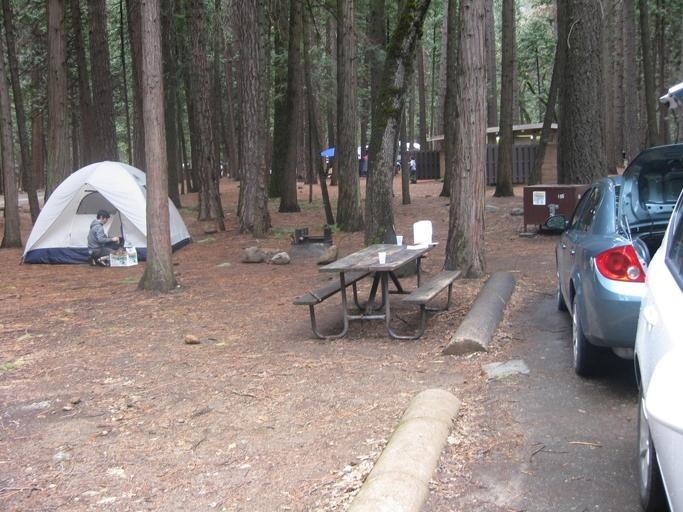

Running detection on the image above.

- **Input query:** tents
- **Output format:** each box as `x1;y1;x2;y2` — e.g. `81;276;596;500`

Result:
16;159;191;266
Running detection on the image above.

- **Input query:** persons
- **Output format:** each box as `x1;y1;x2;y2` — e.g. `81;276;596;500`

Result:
406;155;416;183
85;209;120;267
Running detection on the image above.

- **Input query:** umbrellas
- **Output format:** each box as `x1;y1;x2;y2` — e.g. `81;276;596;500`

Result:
320;148;335;158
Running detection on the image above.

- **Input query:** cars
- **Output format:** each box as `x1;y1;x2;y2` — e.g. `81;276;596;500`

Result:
548;135;682;391
607;75;682;511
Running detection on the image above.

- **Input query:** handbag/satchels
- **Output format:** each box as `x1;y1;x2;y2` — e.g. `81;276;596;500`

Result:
104;236;123;250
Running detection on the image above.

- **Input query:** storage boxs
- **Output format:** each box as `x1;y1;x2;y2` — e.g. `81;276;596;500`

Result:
108;247;139;268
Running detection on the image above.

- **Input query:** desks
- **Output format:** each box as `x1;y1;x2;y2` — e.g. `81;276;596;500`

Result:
310;241;452;339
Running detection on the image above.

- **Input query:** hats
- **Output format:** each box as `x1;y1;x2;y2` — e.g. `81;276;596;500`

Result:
97;210;110;217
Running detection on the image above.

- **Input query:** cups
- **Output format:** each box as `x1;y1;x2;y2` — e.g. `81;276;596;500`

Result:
376;252;388;265
395;235;403;246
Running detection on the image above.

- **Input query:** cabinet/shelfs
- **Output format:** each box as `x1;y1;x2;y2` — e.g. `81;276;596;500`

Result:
524;184;589;232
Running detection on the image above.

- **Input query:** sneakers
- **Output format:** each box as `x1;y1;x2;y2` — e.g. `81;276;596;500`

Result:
89;257;108;267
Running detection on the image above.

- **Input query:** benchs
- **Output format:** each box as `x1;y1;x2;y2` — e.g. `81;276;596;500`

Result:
404;266;462;340
291;271;369;339
